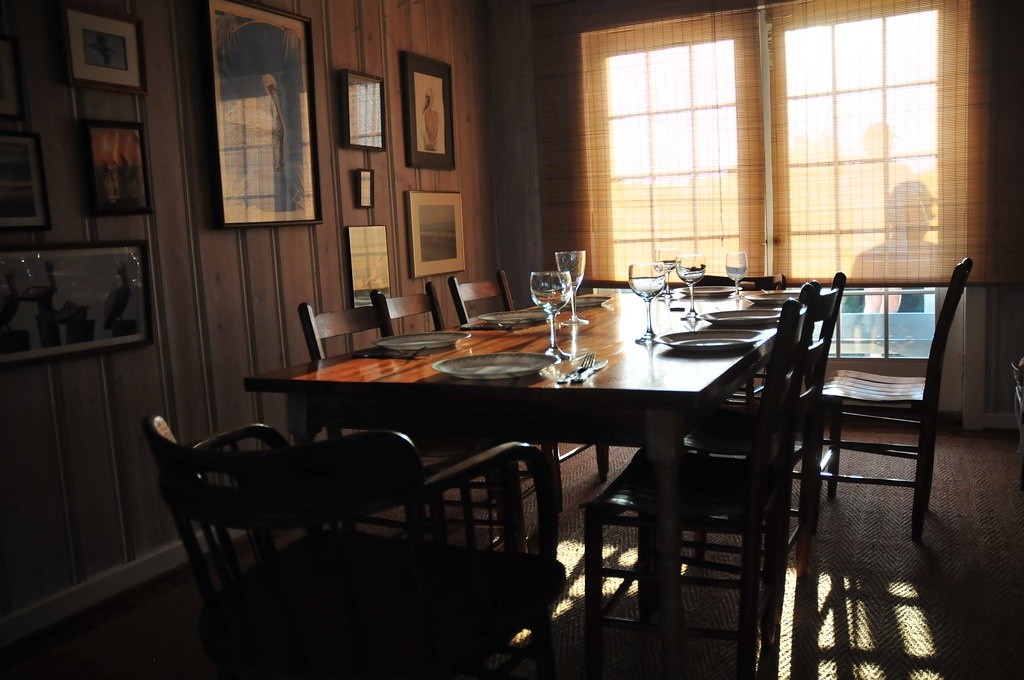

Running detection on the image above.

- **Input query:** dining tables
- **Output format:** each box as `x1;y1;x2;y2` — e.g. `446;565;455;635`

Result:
244;289;801;680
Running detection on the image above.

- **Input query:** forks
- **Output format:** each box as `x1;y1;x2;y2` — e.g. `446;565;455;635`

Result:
556;353;597;384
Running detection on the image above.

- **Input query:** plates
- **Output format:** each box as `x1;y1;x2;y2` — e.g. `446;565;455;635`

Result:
695;308;781;326
370;331;472;351
476;310;548;325
558;296;613;309
744;293;800;305
432;353;561;380
652;330;766;350
673;285;743;297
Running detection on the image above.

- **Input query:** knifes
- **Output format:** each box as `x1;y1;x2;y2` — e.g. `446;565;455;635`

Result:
570;359;608;383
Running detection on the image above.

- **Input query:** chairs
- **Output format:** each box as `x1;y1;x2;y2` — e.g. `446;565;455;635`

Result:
811;257;975;544
143;409;564;680
683;270;846;599
298;268;610;553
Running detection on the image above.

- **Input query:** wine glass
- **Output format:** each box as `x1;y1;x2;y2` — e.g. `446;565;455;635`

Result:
555;250;589;326
675;253;706;321
628;262;665;345
653;248;678;298
725;251;748;298
530;270;572;361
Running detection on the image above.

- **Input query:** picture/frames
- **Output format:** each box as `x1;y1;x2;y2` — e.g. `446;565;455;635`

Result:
0;34;26;120
356;168;375;208
204;0;324;230
344;224;391;308
406;190;467;279
80;115;152;216
0;128;52;231
578;283;815;680
339;68;386;151
400;51;456;172
0;237;156;369
57;0;150;98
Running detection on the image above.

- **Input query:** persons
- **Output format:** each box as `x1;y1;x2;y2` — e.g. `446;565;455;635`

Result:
843;180;937;344
